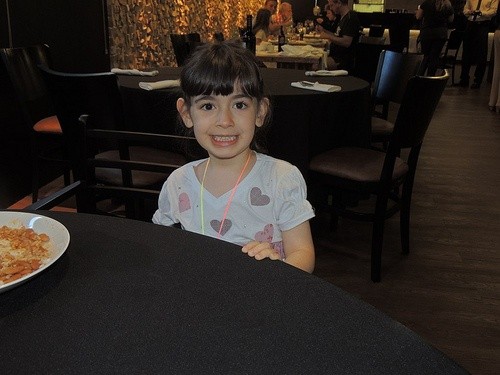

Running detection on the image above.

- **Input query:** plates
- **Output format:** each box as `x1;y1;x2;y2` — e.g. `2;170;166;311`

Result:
0;211;71;295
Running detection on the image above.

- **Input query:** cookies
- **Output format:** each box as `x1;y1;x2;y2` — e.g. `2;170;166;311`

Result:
0;225;50;283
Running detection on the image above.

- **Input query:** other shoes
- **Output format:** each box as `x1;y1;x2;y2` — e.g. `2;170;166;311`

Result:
470;79;481;89
452;79;470;87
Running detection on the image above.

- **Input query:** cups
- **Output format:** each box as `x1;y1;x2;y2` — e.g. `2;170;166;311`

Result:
267;45;274;54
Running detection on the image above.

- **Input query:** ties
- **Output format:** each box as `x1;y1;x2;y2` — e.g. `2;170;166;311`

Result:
473;0;482;21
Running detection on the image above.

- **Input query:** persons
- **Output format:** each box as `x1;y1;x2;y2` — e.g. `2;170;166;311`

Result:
152;41;315;273
253;0;500;114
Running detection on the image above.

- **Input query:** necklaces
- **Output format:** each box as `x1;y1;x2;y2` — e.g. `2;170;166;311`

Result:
201;153;250;238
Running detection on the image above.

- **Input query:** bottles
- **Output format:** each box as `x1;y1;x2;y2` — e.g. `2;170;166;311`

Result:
299;26;303;40
242;15;257;55
306;22;309;34
278;26;285;52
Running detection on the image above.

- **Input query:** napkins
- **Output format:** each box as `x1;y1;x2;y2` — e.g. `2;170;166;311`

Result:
111;68;159;77
139;79;181;91
305;70;349;76
291;81;341;93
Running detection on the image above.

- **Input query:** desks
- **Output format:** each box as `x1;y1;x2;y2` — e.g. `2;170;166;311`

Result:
256;45;326;70
116;68;371;214
1;209;473;375
288;32;321;38
268;36;330;47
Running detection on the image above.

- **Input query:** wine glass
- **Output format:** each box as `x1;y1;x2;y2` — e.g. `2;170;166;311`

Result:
285;20;315;41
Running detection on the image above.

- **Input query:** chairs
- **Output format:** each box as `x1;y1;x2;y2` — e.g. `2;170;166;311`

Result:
0;9;462;283
